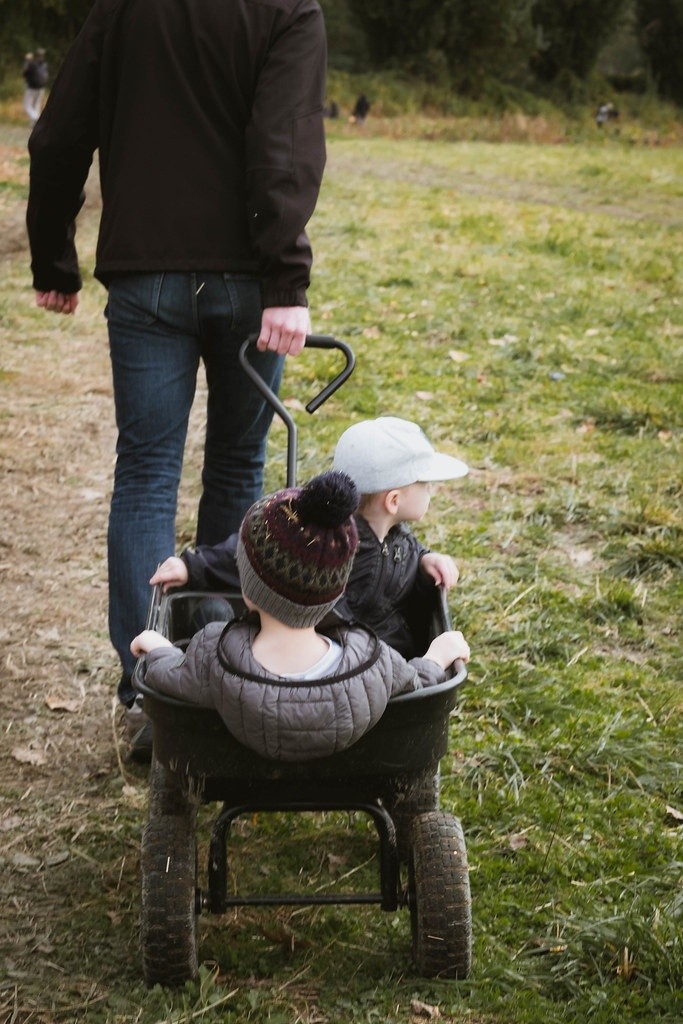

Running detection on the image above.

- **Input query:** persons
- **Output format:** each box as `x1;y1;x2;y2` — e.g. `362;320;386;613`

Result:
21;48;48;125
147;415;470;658
596;101;618;127
26;0;328;759
322;100;337;119
131;471;471;757
353;95;368;128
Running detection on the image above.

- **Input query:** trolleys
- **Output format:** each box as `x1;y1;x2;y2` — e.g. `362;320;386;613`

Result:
137;326;477;983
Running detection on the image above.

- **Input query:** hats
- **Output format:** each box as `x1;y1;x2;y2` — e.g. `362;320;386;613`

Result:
236;471;359;630
332;417;468;494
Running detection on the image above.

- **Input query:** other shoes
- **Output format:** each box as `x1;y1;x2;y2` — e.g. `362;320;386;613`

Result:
112;691;154;785
